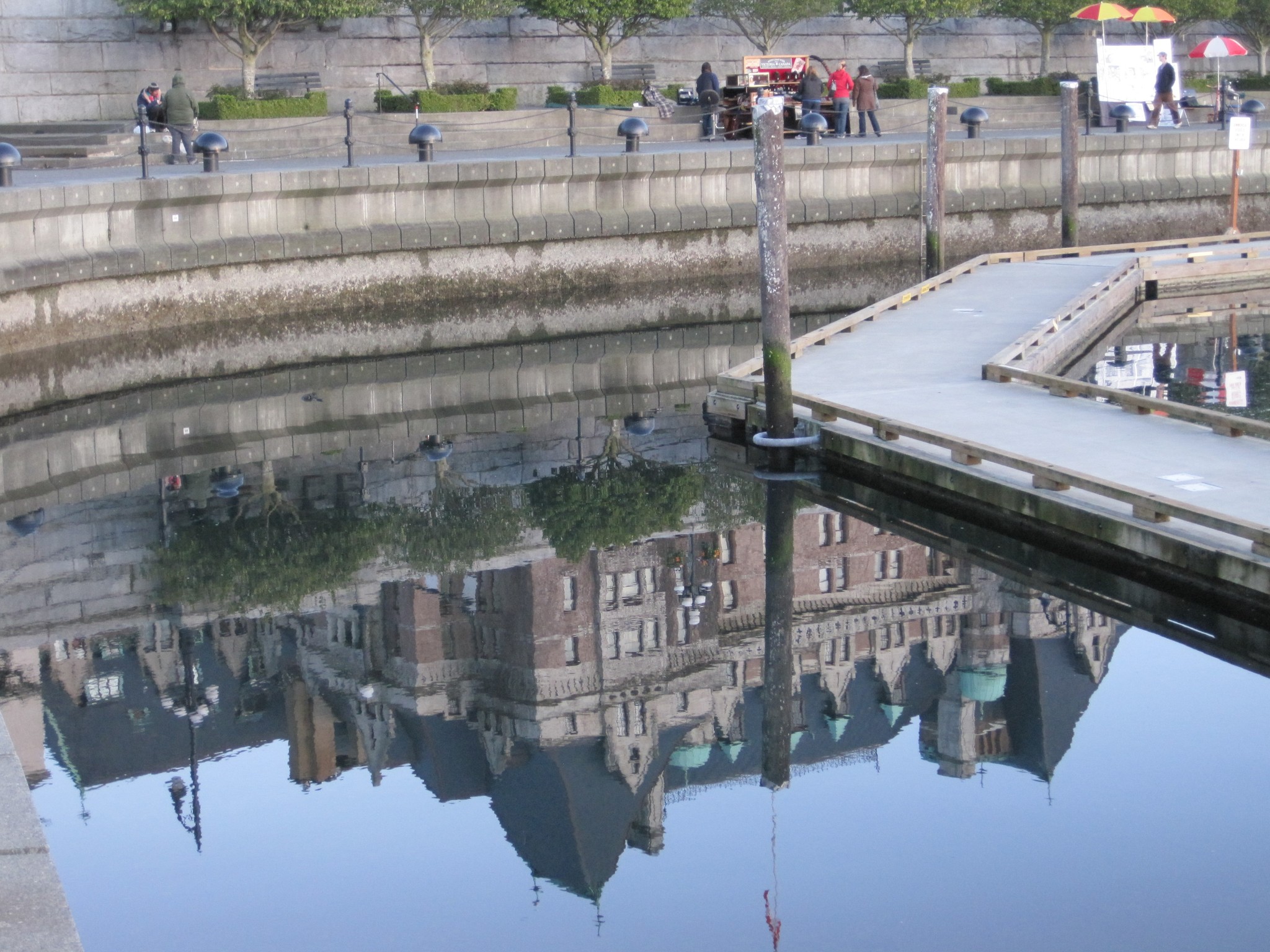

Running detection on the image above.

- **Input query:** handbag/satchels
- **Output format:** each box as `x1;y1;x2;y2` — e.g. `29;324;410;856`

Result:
874;95;880;110
828;74;835;98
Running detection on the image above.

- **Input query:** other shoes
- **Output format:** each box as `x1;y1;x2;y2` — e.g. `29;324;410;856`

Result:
188;159;198;165
855;133;865;138
834;134;845;139
173;160;179;165
795;134;807;139
160;127;169;132
1173;122;1182;128
1146;124;1158;129
150;126;156;132
877;133;882;138
819;136;822;140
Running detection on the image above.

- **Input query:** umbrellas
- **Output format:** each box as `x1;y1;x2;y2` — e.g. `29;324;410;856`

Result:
1069;2;1134;46
1116;6;1176;46
1188;36;1247;111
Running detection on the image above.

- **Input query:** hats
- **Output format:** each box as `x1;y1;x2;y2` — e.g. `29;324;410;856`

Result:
1156;51;1167;58
148;83;158;92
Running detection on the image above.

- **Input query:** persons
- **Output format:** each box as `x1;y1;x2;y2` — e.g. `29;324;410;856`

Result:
696;62;719;142
137;72;170;133
162;73;199;165
852;65;882;138
1147;52;1183;129
826;60;854;139
794;65;825;140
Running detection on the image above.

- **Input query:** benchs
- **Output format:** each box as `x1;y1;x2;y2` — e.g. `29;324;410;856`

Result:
591;64;666;88
254;72;322;100
877;59;932;77
